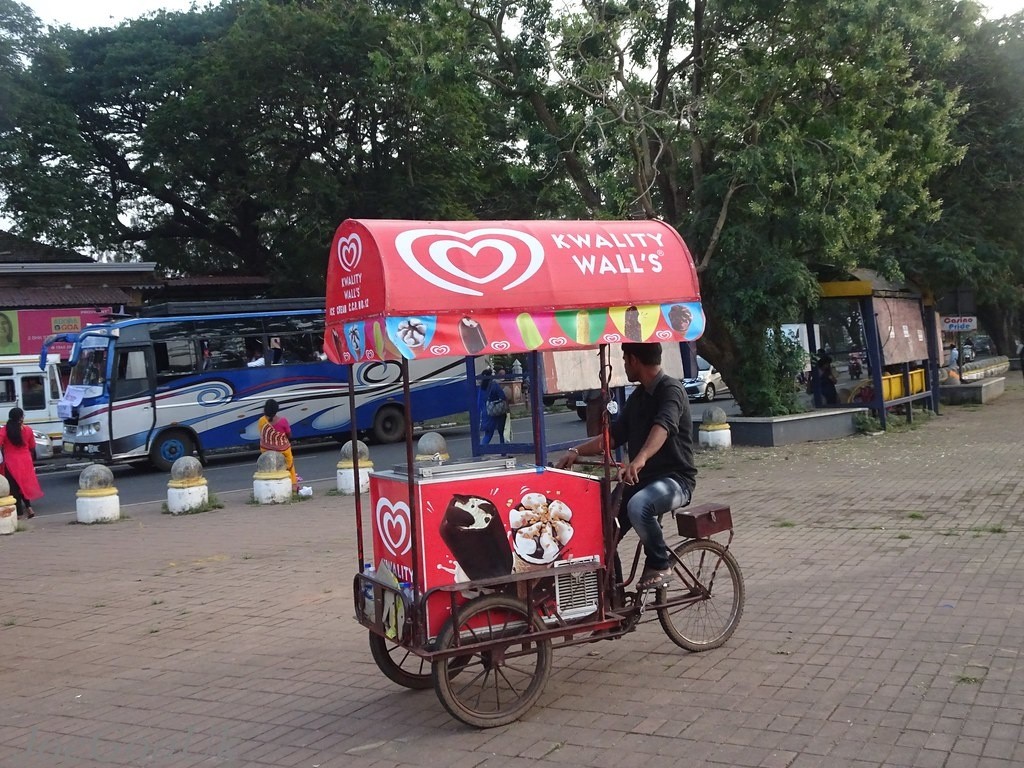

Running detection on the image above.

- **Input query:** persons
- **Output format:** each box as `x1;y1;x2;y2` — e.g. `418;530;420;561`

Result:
54;324;78;330
809;349;841;406
554;338;696;608
849;357;861;375
949;342;959;367
249;346;264;363
0;408;45;521
796;372;808;385
1015;337;1024;378
479;369;509;457
91;360;104;378
824;342;831;354
882;360;918;415
0;313;18;354
24;377;44;401
258;400;301;493
964;337;973;346
315;341;327;361
498;369;506;375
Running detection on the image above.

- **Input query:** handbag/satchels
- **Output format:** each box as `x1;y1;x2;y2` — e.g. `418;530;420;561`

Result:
485;382;507;417
503;413;513;444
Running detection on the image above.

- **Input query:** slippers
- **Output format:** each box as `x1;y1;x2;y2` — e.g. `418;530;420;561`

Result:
589;627;620;644
636;567;676;591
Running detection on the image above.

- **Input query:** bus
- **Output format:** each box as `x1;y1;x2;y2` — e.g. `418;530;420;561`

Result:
39;298;493;474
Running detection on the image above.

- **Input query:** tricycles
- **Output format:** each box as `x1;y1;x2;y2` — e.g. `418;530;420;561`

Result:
320;217;745;729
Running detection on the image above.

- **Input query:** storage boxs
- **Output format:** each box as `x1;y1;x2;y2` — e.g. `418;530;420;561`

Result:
676;502;733;538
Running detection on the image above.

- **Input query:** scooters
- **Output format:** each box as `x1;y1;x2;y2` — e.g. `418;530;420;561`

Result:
962;344;975;363
847;361;860;380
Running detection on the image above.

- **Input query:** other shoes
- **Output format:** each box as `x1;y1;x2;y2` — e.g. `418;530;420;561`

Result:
293;485;301;492
27;512;34;518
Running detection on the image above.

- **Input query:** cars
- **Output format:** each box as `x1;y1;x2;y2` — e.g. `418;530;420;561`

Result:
679;354;729;403
0;423;53;460
566;384;638;424
972;334;993;357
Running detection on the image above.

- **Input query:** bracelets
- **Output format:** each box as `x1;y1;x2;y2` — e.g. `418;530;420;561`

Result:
568;447;579;455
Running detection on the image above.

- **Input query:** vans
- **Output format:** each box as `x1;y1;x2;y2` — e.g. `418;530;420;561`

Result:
0;354;66;448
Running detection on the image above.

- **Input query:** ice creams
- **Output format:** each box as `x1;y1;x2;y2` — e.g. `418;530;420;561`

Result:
508;493;573;600
397;320;426;357
349;325;362;361
668;306;693;337
